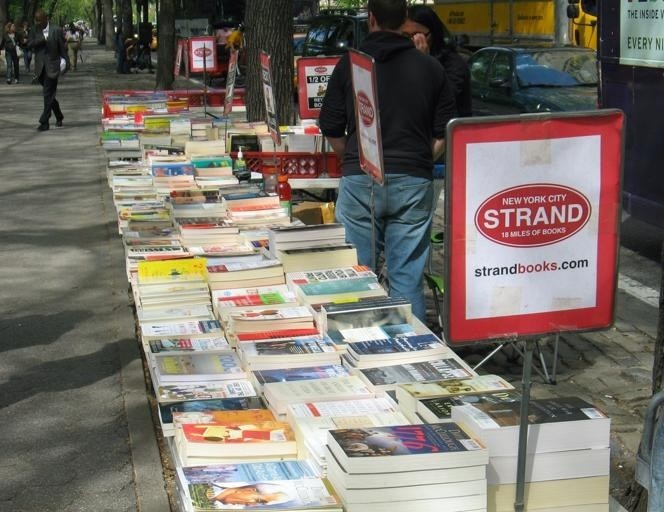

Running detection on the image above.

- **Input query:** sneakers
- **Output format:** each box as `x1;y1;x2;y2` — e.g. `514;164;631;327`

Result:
7;78;19;84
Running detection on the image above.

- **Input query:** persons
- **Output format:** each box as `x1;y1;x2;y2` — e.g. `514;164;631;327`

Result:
401;3;473;213
0;9;89;132
114;21;155;74
318;0;459;330
215;22;245;65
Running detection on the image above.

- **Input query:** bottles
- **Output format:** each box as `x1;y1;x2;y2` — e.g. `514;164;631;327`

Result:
275;175;294;221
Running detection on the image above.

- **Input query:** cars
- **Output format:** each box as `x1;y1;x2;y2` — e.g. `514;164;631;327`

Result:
466;45;600;116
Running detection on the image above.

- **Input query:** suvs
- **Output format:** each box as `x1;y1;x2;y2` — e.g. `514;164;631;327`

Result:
291;9;370;95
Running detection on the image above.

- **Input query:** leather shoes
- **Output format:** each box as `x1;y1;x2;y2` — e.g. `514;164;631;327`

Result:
37;122;49;132
54;115;64;127
